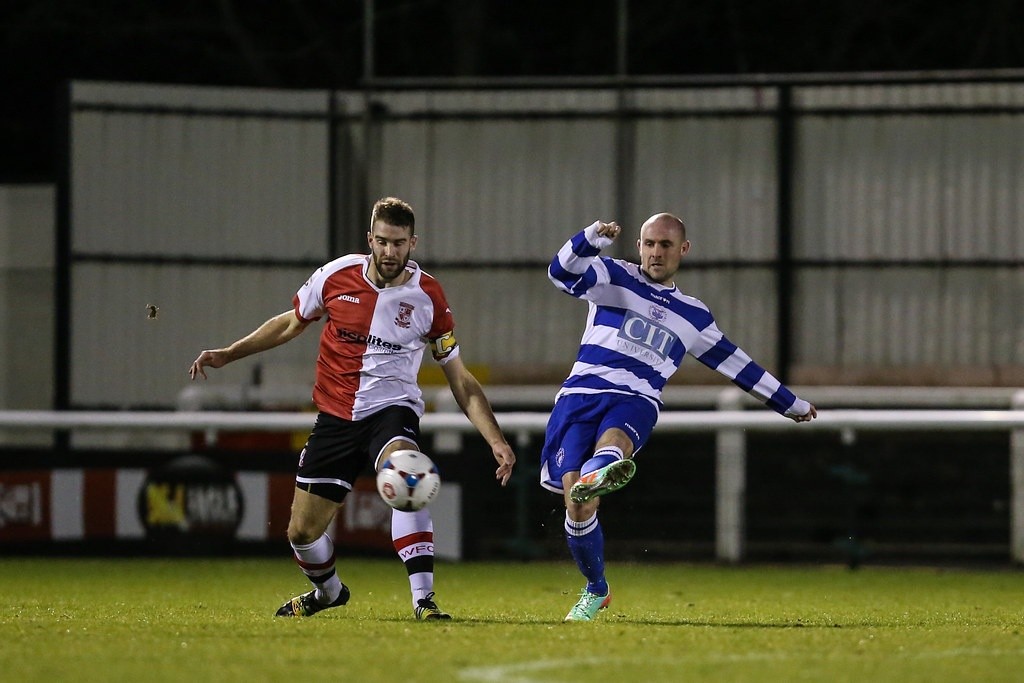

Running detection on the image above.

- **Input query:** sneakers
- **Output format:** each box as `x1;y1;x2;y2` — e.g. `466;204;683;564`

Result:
564;581;611;623
414;591;451;622
276;582;351;618
569;459;636;503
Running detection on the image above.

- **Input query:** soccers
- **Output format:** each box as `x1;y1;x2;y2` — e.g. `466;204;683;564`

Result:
377;449;440;513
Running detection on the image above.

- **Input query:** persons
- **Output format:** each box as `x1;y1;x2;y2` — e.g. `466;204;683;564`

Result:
541;213;817;620
189;198;516;620
